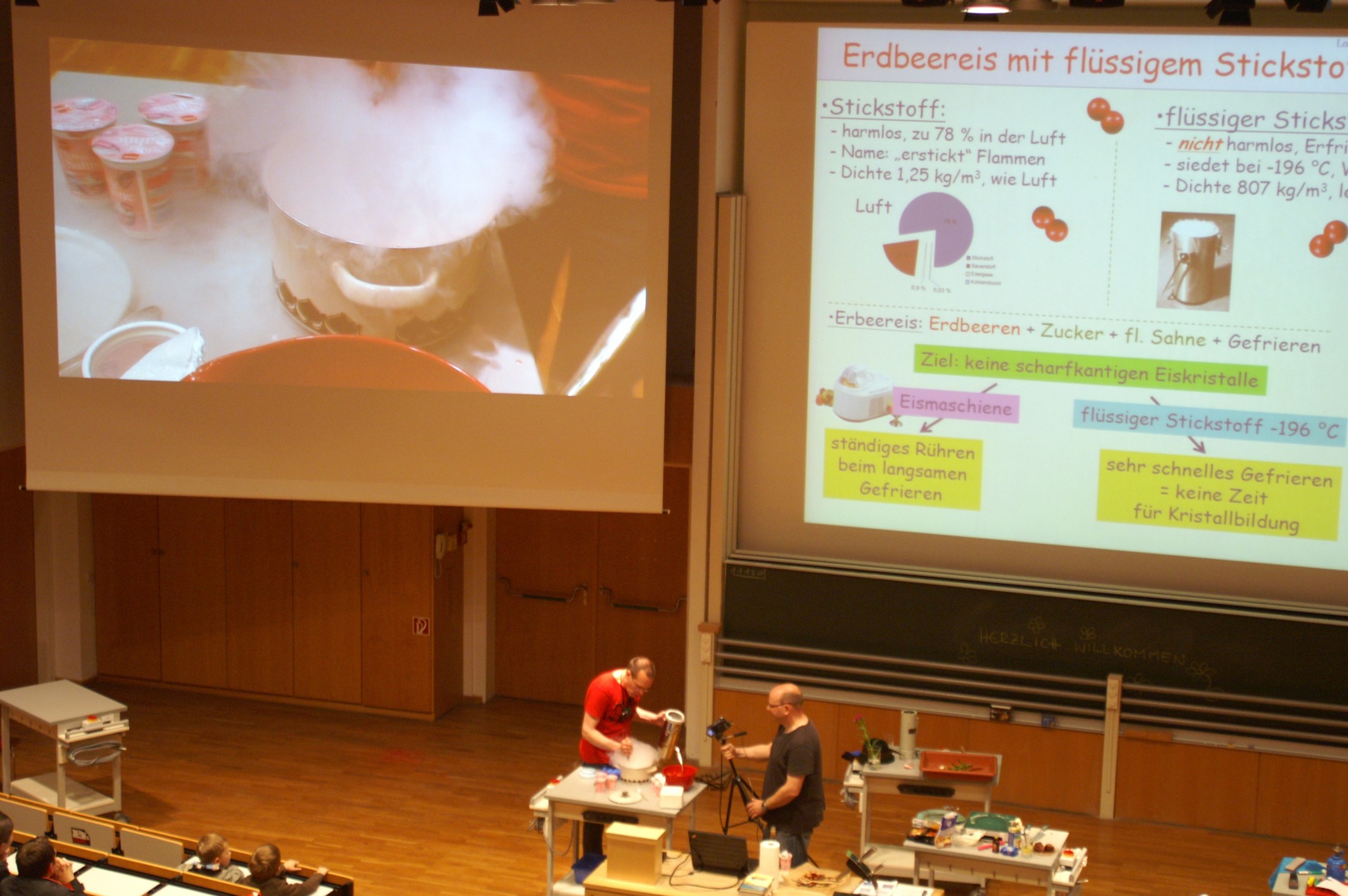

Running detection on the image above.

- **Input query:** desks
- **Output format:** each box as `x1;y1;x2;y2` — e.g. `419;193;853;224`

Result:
529;766;708;896
844;746;1002;858
861;843;989;888
1272;857;1348;896
583;846;945;896
903;826;1088;896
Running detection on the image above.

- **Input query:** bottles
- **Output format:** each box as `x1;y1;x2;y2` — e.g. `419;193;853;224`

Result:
992;836;999;853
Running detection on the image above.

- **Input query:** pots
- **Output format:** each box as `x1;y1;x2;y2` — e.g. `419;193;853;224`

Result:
616;755;657;781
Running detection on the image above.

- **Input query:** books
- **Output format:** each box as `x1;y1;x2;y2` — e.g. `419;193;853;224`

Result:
852;880;933;896
737;872;774;896
964;811;1023;842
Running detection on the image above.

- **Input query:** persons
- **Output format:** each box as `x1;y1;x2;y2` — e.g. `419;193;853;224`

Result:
0;836;87;896
720;682;826;868
180;833;245;884
578;657;666;857
0;810;17;885
239;844;329;896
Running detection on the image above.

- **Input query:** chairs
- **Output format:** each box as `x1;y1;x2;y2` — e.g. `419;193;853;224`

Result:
0;792;354;896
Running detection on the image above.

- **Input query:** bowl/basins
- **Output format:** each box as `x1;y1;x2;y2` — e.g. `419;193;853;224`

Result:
662;765;698;792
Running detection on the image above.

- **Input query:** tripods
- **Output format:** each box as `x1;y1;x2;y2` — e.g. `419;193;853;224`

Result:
715;731;768;842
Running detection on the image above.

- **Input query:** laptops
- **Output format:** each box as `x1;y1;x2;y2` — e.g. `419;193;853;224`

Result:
688;830;758;878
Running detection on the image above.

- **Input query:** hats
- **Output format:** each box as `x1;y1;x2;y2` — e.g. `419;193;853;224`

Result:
858;738;895;765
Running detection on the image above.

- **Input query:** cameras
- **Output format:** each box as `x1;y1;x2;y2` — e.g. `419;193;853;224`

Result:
705;717;731;738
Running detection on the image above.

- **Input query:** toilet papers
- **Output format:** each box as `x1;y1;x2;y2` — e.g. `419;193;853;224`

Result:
758;839;780;876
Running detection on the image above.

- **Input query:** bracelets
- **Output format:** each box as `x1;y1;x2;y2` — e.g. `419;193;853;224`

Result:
762;802;770;812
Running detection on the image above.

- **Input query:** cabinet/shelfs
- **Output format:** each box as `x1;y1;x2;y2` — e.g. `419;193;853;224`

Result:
606;821;666;886
0;678;130;824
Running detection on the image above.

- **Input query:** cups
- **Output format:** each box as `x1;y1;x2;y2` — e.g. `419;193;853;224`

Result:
780;854;792;871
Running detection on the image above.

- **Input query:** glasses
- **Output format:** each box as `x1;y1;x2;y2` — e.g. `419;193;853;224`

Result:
632;678;650;692
767;702;792;707
